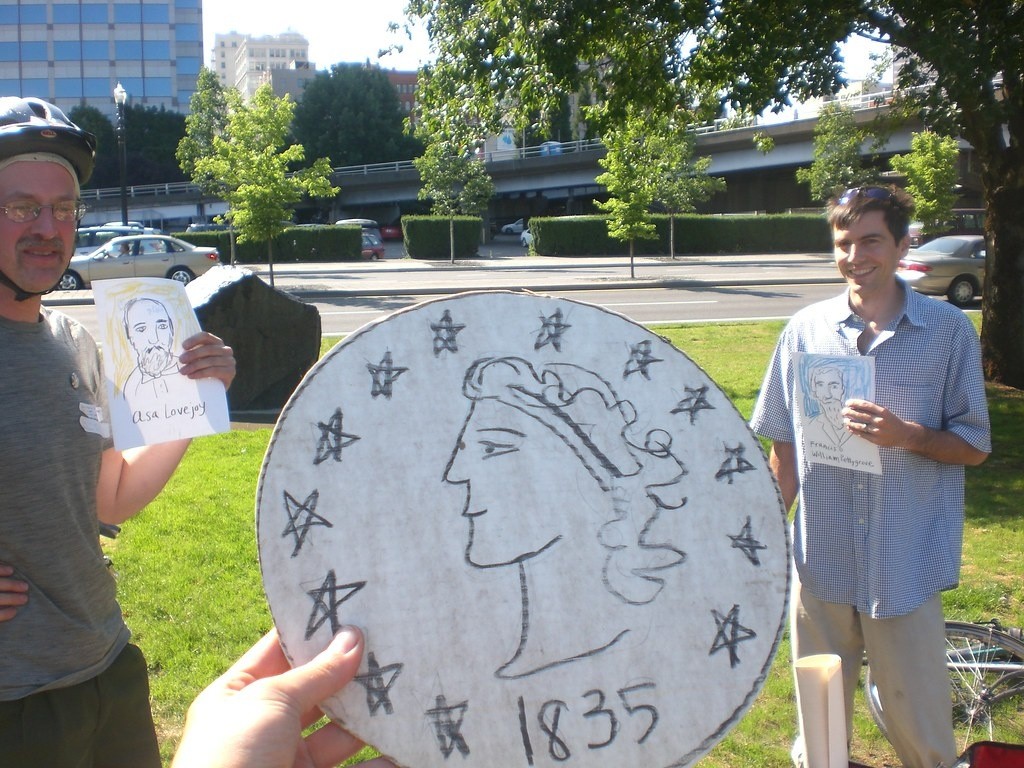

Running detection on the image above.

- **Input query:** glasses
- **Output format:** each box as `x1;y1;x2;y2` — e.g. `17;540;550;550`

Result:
1;199;93;223
838;186;909;214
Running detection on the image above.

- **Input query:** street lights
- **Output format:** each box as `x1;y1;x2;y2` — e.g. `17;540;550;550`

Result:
113;81;129;236
521;114;527;158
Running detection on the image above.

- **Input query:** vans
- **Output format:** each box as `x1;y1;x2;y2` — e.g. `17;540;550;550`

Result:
906;208;990;248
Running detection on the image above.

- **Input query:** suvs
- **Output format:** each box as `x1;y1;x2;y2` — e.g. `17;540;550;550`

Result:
187;223;236;233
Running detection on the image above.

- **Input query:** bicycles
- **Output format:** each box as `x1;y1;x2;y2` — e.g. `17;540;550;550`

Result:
862;618;1024;759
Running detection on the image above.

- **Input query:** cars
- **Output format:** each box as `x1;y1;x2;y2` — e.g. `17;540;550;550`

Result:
55;236;220;291
501;218;524;234
521;230;534;246
381;219;405;241
75;222;165;252
895;235;986;306
281;219;386;262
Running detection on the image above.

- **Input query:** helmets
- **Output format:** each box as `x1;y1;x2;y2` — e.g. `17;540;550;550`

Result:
1;96;97;195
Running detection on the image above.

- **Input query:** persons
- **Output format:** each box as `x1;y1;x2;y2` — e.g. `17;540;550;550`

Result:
169;625;398;768
748;185;992;768
0;96;237;768
119;243;130;256
